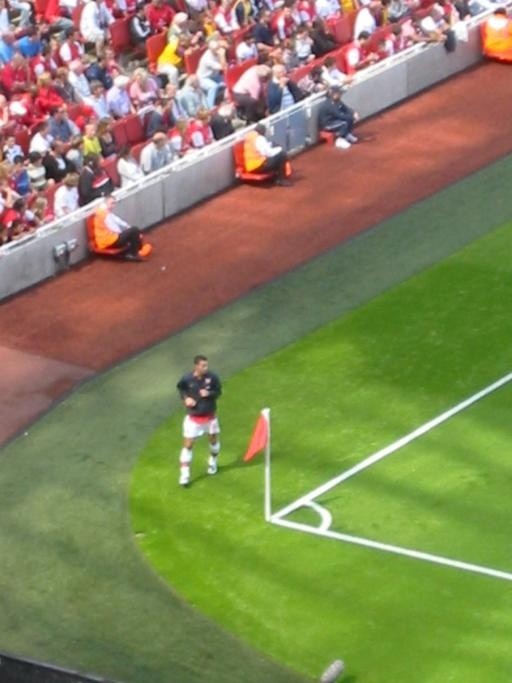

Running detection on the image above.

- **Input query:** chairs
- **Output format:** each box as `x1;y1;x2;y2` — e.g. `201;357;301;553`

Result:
0;0;442;243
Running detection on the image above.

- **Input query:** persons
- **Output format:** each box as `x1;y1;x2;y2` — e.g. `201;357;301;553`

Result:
174;354;222;486
91;193;145;263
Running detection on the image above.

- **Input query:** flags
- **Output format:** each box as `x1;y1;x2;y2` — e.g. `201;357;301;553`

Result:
241;408;269;464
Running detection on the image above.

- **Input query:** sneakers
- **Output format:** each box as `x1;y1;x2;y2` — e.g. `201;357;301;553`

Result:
206;454;219;476
335;133;359;149
179;466;190;486
